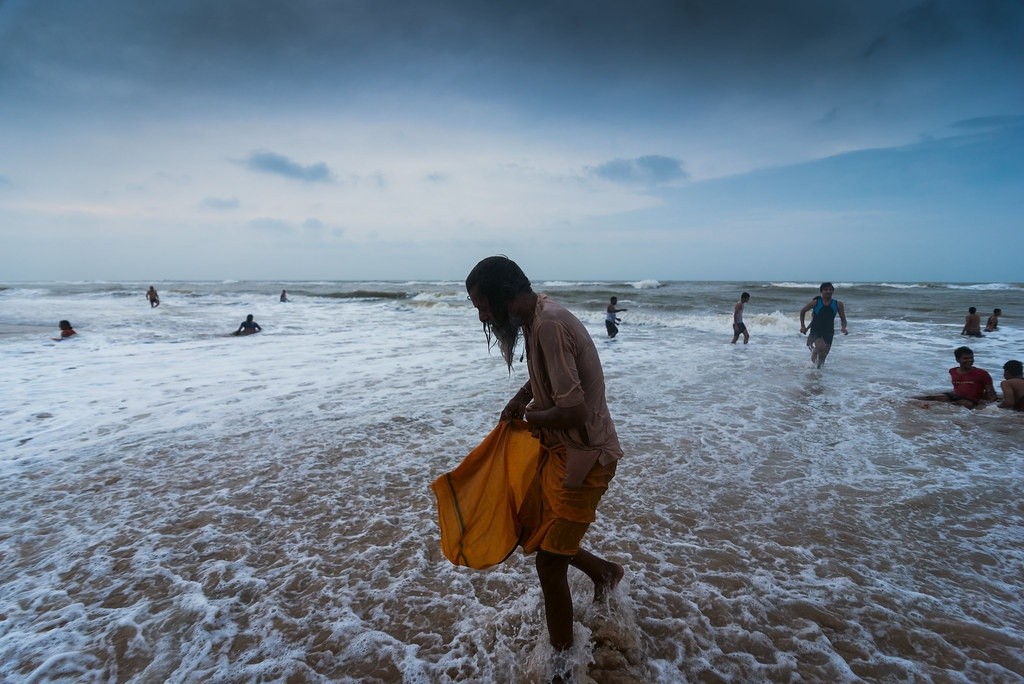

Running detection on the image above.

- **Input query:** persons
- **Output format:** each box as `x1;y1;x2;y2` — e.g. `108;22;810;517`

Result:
234;314;263;337
912;346;998;409
983;308;1002;332
605;296;628;337
801;281;850;369
280;289;290;303
961;306;981;336
998;359;1024;412
145;285;160;309
730;291;751;344
58;319;80;338
467;255;625;682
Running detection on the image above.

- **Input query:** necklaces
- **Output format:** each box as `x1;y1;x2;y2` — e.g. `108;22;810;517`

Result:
520;327;537;362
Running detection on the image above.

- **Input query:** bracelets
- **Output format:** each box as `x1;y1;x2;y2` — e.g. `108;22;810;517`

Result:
520;386;533;400
